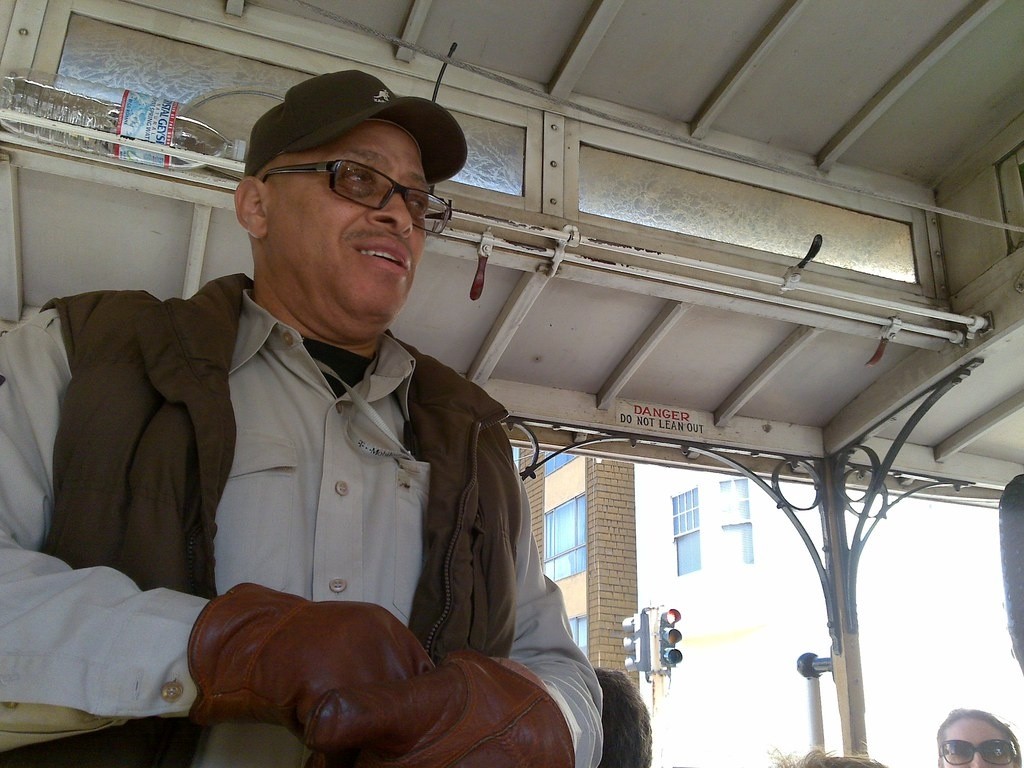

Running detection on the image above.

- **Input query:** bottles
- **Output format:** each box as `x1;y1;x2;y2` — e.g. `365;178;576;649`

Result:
2;66;247;170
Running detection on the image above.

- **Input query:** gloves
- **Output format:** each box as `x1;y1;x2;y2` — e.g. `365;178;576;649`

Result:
304;647;574;768
187;581;436;743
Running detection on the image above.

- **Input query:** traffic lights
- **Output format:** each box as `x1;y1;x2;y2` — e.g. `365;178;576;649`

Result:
647;605;683;672
622;615;648;672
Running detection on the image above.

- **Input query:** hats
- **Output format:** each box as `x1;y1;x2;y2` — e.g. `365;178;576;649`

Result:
244;69;467;185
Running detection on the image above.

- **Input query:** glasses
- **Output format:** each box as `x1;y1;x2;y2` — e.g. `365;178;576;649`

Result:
940;738;1018;765
261;158;453;234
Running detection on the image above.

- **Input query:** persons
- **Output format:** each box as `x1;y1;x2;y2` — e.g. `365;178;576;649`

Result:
768;747;887;768
937;708;1022;768
0;70;604;768
594;667;653;768
